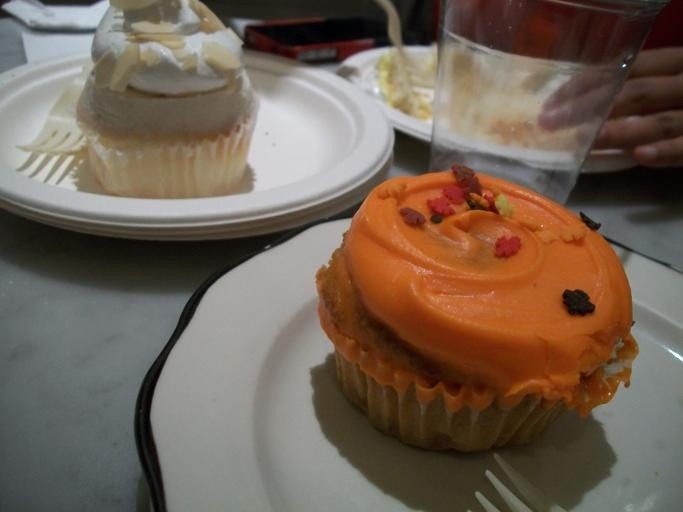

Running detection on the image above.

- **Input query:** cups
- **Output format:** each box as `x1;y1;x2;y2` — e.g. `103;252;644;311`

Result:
429;1;659;205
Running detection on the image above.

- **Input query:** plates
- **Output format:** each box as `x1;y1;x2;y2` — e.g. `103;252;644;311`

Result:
145;216;682;511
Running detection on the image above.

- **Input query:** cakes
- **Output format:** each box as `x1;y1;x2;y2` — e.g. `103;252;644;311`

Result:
88;0;259;197
315;163;640;454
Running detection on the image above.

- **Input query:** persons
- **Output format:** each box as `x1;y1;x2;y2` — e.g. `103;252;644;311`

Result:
539;47;683;168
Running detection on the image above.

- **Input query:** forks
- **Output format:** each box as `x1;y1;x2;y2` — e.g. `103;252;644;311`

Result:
13;72;93;158
